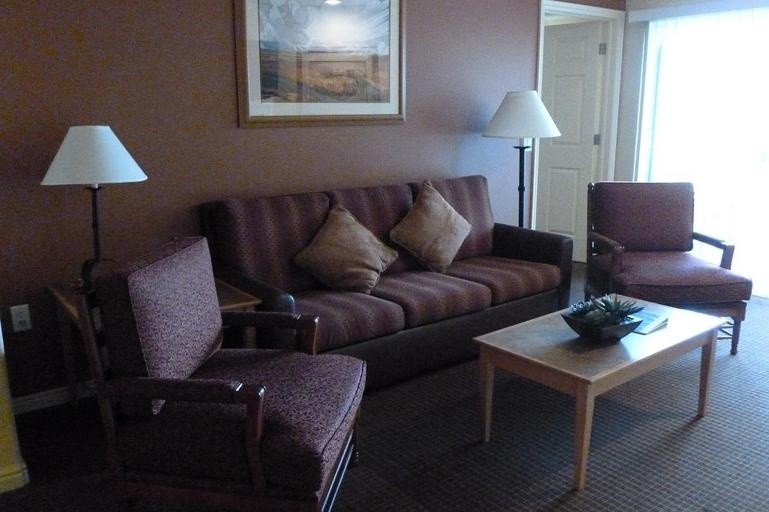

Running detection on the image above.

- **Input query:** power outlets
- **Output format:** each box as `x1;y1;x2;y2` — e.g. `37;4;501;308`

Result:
9;304;32;332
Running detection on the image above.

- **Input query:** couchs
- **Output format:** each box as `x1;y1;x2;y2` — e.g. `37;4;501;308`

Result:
194;177;572;396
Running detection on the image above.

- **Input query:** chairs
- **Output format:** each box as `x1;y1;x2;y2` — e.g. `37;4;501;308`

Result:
584;181;752;355
81;260;366;512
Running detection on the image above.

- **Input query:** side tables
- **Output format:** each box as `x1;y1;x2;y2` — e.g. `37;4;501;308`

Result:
47;274;263;366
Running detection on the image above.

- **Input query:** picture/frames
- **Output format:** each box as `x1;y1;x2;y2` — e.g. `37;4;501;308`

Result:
232;0;406;129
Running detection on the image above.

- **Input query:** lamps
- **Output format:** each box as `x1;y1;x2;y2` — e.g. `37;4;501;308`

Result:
481;89;562;230
40;123;149;273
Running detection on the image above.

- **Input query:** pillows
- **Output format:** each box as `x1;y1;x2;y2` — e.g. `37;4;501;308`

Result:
297;203;398;294
94;235;223;415
389;179;473;273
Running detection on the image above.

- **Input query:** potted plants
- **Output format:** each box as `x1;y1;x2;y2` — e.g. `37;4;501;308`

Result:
561;293;644;346
